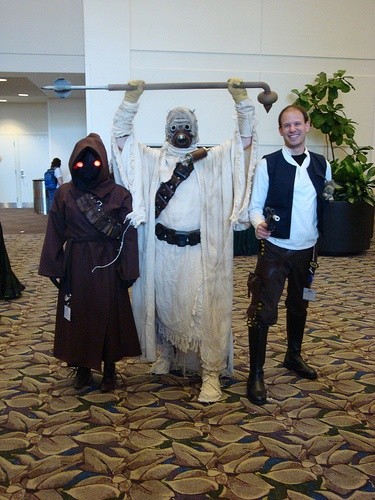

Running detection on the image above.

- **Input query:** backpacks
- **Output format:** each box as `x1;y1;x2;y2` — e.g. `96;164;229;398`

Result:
44;167;58;189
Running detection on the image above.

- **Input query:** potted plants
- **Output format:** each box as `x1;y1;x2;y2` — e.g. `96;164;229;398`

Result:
290;69;375;257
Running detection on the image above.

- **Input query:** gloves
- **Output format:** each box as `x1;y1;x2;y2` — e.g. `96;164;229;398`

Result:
227;77;247;103
124;80;144;103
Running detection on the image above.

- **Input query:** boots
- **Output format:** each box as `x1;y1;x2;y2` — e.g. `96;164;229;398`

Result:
246;328;268;405
284;312;317;380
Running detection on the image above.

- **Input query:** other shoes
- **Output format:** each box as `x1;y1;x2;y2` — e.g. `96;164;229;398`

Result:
100;363;115;393
198;375;221;403
0;293;21;300
152;359;169;374
74;367;97;392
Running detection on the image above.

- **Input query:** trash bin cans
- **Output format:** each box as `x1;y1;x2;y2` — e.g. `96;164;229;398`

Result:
32;178;46;215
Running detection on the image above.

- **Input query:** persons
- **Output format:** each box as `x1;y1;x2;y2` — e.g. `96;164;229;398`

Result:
49;158;63;203
109;79;258;404
246;106;332;406
38;133;143;392
0;222;25;299
